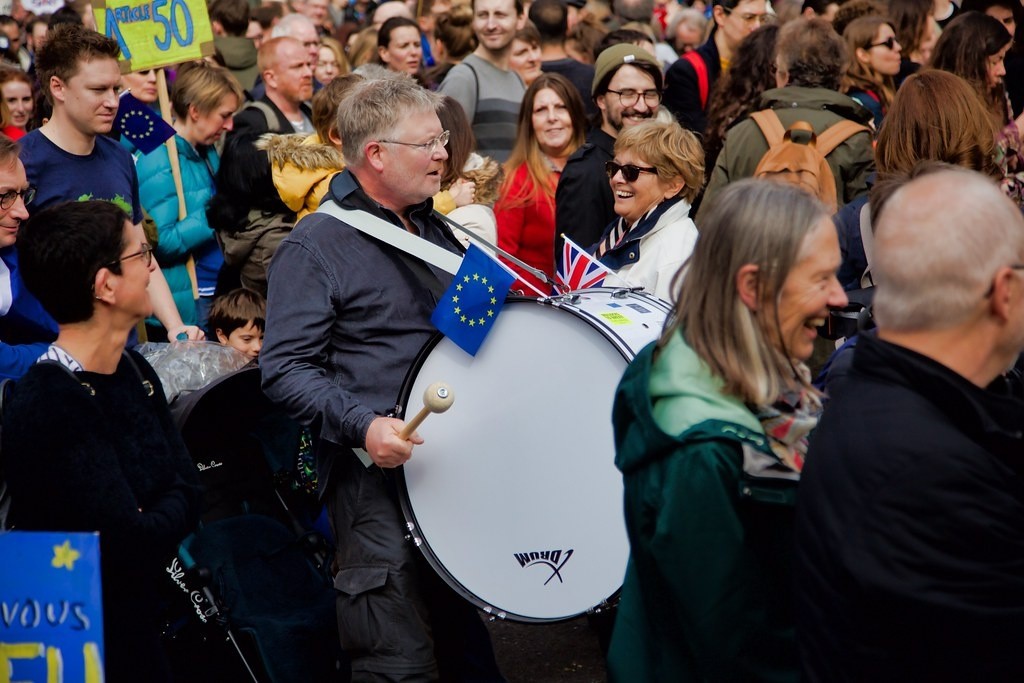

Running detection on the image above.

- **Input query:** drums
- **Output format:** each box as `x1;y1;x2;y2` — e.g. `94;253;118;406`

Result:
392;285;677;626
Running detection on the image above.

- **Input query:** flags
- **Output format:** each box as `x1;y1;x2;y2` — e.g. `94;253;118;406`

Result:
0;531;102;683
429;241;518;359
551;237;612;299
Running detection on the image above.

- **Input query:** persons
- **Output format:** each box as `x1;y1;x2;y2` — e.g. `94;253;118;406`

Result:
0;1;1024;683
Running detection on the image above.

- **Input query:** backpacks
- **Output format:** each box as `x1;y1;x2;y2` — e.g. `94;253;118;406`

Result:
0;349;154;531
750;109;874;218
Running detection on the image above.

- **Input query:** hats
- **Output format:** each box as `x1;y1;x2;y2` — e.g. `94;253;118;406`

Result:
592;43;664;102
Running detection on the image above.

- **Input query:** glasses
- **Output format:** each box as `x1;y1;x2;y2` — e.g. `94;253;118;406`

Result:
92;243;152;288
722;6;773;25
377;130;449;155
863;37;901;50
605;88;663;108
0;190;36;210
604;160;658;182
